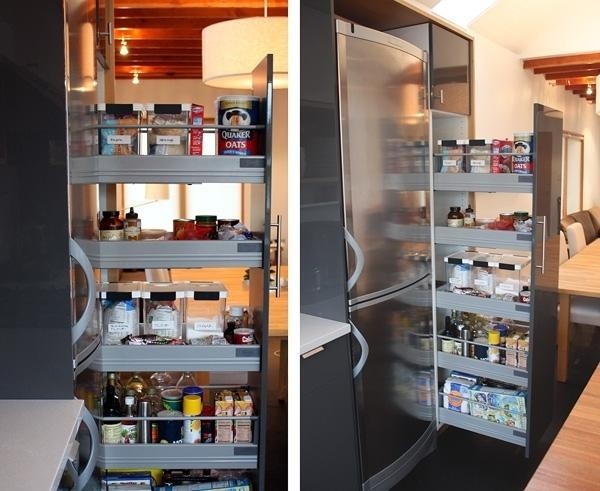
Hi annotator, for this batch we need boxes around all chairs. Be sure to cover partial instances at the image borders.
[559,203,600,382]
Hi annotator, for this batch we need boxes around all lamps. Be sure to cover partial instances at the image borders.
[202,0,289,90]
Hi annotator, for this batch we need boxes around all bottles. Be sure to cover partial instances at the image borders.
[500,212,527,231]
[103,373,213,442]
[101,207,141,241]
[442,311,508,366]
[195,215,216,239]
[447,204,477,227]
[228,306,254,345]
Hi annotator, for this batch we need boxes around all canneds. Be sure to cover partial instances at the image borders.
[173,219,194,240]
[234,329,254,344]
[514,213,528,220]
[218,219,239,231]
[500,213,512,222]
[196,216,217,232]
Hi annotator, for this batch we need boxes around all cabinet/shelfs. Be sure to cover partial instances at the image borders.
[432,102,562,460]
[71,52,274,491]
